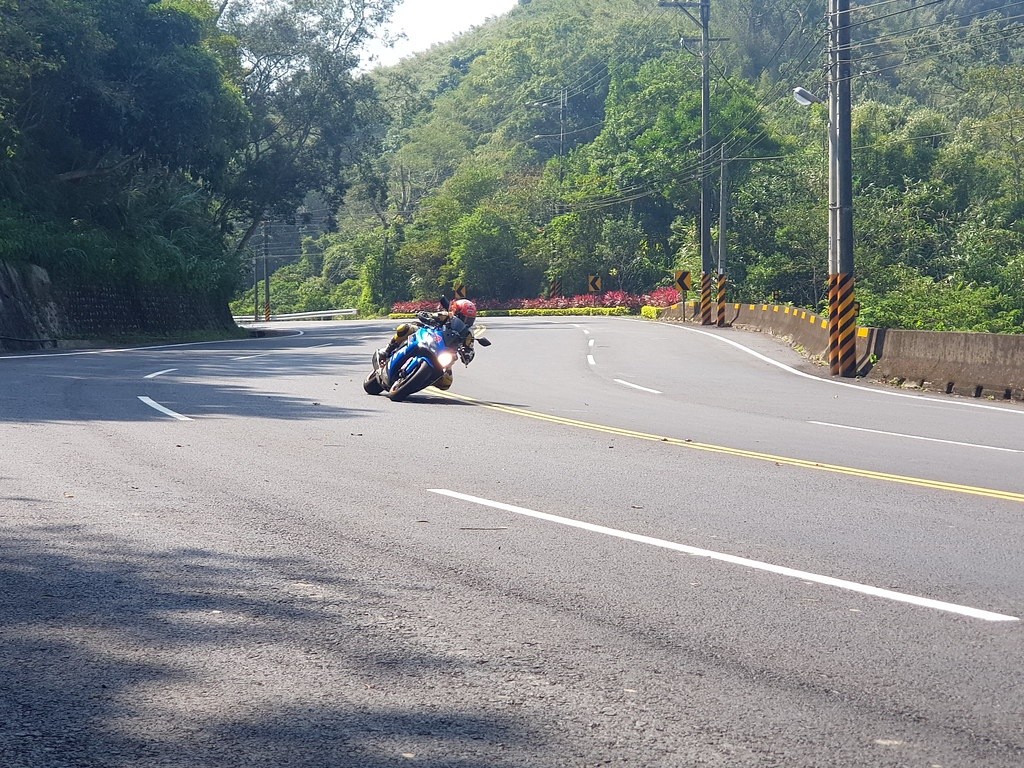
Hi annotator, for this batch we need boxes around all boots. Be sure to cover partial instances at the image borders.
[379,338,401,359]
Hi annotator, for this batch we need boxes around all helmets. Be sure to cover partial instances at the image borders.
[448,298,476,327]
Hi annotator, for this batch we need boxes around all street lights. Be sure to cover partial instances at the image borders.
[792,87,839,378]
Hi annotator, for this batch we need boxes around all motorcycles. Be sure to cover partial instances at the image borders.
[363,294,491,402]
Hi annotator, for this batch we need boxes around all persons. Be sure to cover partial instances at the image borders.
[380,298,478,390]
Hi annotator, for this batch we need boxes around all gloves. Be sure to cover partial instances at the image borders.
[461,351,473,364]
[417,310,431,322]
